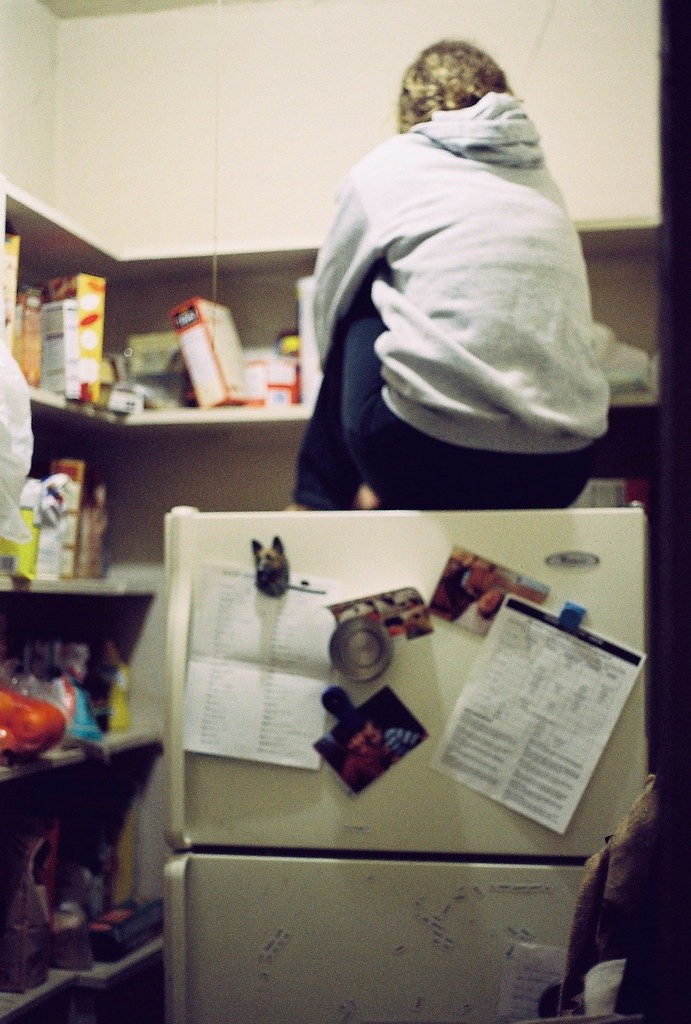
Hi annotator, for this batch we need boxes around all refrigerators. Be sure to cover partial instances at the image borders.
[146,503,654,1024]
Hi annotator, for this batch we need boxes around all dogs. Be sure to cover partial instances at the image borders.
[252,536,288,596]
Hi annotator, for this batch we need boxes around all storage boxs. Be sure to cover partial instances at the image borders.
[88,895,164,964]
[169,296,252,409]
[50,455,86,578]
[43,273,107,402]
[40,298,83,400]
[0,233,21,350]
[572,479,652,517]
[243,357,301,406]
[12,286,41,388]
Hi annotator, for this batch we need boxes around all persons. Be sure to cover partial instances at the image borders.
[289,42,613,510]
[442,564,503,635]
[339,719,424,792]
[0,339,35,543]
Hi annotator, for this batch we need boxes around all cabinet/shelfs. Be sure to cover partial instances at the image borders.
[0,181,669,1024]
